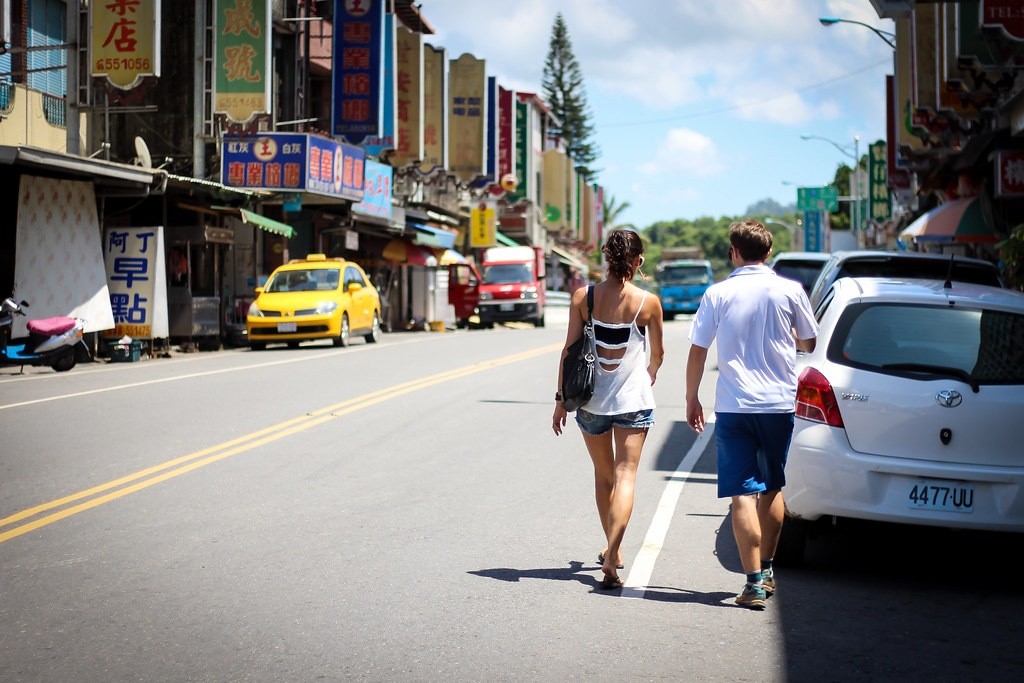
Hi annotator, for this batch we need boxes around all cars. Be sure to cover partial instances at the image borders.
[776,277,1024,609]
[769,251,832,298]
[246,253,382,350]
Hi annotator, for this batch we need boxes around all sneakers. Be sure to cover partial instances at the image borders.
[736,584,766,608]
[763,570,776,594]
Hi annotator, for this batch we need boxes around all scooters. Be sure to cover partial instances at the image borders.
[0,290,92,375]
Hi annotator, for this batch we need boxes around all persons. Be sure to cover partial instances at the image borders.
[552,230,664,588]
[686,218,821,609]
[327,272,339,288]
[165,245,182,291]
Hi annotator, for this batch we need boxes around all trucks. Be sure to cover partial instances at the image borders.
[448,246,547,329]
[654,260,714,322]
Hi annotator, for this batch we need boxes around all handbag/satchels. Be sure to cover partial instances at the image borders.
[562,285,595,412]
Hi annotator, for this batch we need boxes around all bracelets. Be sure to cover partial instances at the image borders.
[554,393,562,401]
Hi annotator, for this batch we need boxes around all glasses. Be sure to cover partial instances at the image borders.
[729,247,732,261]
[639,255,644,267]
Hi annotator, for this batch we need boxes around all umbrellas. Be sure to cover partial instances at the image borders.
[897,197,1024,244]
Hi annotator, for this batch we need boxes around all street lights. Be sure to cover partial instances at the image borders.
[801,133,863,250]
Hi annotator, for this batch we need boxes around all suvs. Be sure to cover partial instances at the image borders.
[808,249,1006,316]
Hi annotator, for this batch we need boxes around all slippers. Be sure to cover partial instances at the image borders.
[600,576,621,589]
[598,550,624,569]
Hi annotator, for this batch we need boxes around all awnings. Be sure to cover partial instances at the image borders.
[0,143,256,206]
[209,206,299,240]
[406,225,457,250]
